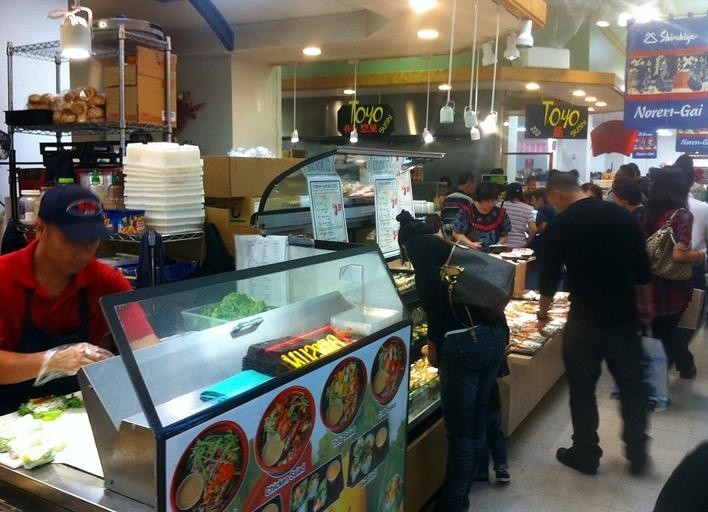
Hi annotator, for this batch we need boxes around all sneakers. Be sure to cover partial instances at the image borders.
[495,469,510,482]
[472,475,489,483]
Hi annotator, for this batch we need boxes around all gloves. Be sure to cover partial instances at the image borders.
[33,343,115,387]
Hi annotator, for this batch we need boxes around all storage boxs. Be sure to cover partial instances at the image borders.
[201,156,308,198]
[106,46,179,129]
[205,198,304,257]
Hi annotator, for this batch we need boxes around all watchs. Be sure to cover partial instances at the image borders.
[536,312,549,320]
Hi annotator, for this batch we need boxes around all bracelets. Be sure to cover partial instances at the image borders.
[697,248,707,264]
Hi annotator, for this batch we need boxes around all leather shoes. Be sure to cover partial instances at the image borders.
[679,365,696,381]
[625,435,652,469]
[557,443,603,476]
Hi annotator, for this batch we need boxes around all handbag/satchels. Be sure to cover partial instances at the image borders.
[403,235,516,338]
[635,209,693,281]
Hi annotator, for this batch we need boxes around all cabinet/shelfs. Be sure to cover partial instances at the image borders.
[386,257,565,512]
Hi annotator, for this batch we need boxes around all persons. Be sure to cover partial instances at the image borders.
[524,190,558,231]
[396,212,507,512]
[675,154,707,199]
[441,174,472,232]
[535,170,655,474]
[0,186,161,414]
[612,178,671,412]
[472,329,510,483]
[498,182,535,249]
[667,164,707,251]
[643,165,708,379]
[449,184,510,252]
[610,161,648,204]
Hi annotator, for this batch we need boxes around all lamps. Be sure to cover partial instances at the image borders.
[440,0,457,124]
[515,20,534,49]
[463,0,479,129]
[290,62,300,143]
[482,41,498,66]
[422,71,434,144]
[349,58,358,144]
[470,45,481,141]
[503,35,520,61]
[482,8,500,134]
[46,0,99,61]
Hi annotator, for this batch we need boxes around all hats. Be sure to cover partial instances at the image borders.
[38,184,111,242]
[506,183,522,194]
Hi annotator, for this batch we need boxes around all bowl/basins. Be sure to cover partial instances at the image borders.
[502,256,519,262]
[181,303,231,330]
[593,179,613,188]
[123,142,206,237]
[253,386,316,478]
[3,109,54,126]
[518,254,530,260]
[489,245,507,252]
[170,421,249,512]
[320,357,368,433]
[371,337,407,405]
